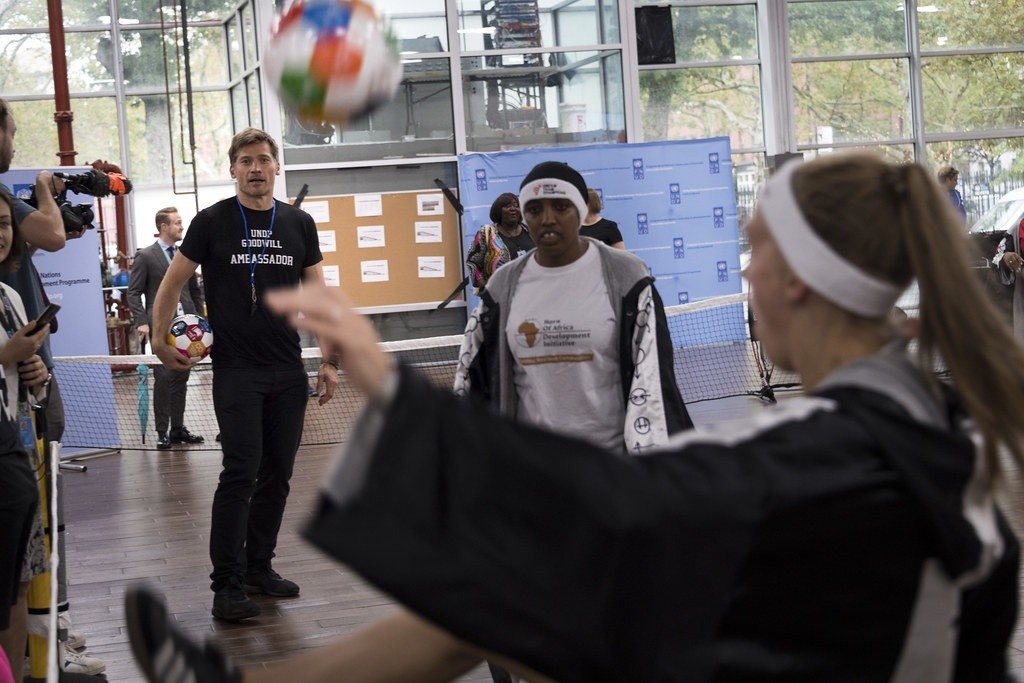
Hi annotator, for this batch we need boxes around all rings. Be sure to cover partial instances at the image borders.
[1009,261,1012,263]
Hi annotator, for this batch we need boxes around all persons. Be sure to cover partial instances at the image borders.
[150,128,340,620]
[453,161,697,454]
[125,206,205,449]
[578,188,625,249]
[0,99,107,683]
[465,193,537,296]
[119,148,1024,683]
[937,166,967,220]
[991,212,1024,342]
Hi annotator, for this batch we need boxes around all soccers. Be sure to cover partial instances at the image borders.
[166,313,214,363]
[264,0,405,125]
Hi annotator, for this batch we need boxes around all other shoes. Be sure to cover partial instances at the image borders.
[216,435,222,441]
[308,386,319,397]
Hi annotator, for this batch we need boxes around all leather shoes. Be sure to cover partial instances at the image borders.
[156,431,171,448]
[169,426,204,443]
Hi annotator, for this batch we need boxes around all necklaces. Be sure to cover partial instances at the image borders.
[235,194,276,302]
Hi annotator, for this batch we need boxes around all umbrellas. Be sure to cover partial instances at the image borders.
[135,333,150,444]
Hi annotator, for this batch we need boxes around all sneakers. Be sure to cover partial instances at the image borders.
[243,570,299,596]
[211,593,265,621]
[20,655,33,676]
[64,633,87,649]
[125,581,243,683]
[57,639,107,674]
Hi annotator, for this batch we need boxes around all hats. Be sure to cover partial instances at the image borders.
[520,161,589,230]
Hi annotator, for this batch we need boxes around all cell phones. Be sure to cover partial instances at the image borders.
[25,303,61,336]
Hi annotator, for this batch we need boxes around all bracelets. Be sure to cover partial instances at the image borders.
[321,358,340,371]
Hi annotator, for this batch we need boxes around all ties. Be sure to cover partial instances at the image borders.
[166,246,175,261]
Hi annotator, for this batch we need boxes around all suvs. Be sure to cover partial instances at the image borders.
[968,186,1024,330]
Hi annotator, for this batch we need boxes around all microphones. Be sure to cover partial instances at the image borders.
[52,172,133,198]
